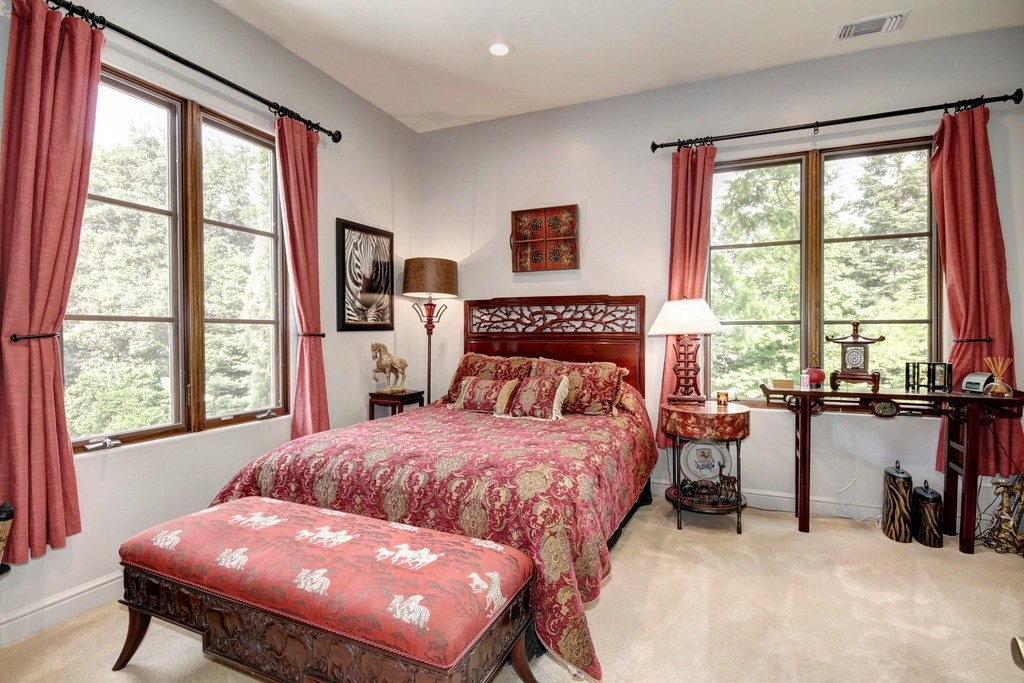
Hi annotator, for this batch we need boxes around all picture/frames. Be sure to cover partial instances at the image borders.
[336,218,394,331]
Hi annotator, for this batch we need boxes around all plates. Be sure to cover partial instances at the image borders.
[681,440,732,485]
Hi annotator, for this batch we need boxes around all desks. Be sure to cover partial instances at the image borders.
[368,390,425,420]
[760,383,1024,554]
[658,402,751,531]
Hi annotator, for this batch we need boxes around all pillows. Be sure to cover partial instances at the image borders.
[536,356,630,416]
[511,375,569,421]
[453,376,518,414]
[448,352,539,406]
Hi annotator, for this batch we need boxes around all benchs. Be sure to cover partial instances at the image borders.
[111,494,539,683]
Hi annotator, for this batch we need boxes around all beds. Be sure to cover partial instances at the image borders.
[204,295,658,683]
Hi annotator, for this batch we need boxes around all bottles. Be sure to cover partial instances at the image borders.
[800,370,810,391]
[985,377,1014,396]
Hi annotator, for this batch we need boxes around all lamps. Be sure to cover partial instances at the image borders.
[402,257,458,405]
[647,296,724,406]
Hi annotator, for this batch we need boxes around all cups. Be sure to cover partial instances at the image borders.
[718,391,728,407]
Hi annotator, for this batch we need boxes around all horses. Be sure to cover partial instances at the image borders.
[369,340,409,388]
